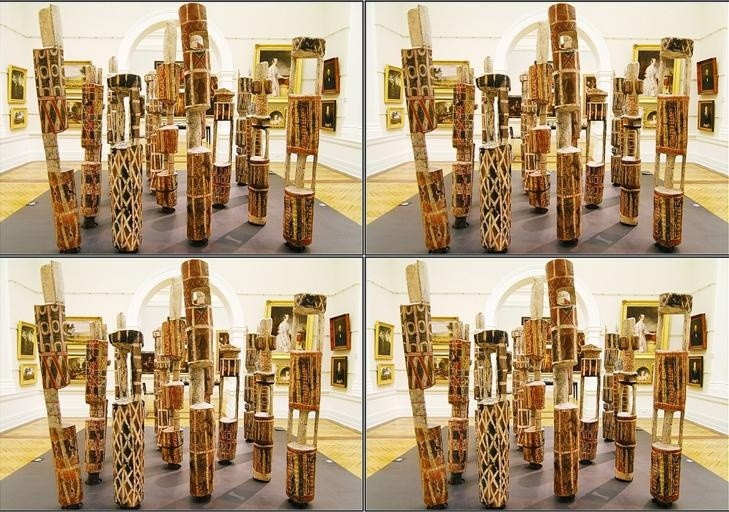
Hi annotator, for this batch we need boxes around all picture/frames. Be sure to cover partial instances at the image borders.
[375,316,462,387]
[266,299,352,390]
[18,317,104,385]
[251,44,340,131]
[7,60,93,130]
[617,300,707,387]
[384,61,475,130]
[631,44,718,132]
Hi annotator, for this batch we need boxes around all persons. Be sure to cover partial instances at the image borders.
[690,361,700,384]
[336,323,346,345]
[637,369,648,381]
[701,104,712,128]
[21,330,33,354]
[276,314,292,351]
[324,105,331,127]
[334,361,343,383]
[691,324,703,347]
[12,73,23,99]
[702,68,713,90]
[324,68,334,88]
[643,58,658,97]
[378,330,390,355]
[268,56,288,97]
[282,369,291,381]
[633,313,655,353]
